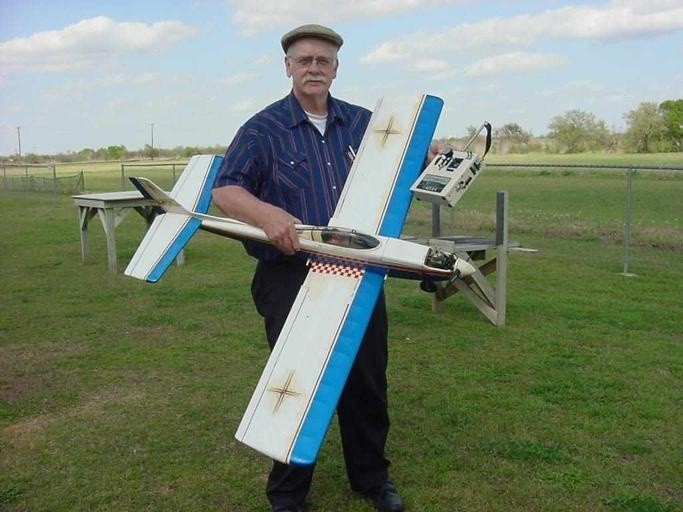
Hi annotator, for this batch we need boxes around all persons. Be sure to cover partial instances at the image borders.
[205,23,463,511]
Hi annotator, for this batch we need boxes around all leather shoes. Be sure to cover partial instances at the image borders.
[367,475,404,512]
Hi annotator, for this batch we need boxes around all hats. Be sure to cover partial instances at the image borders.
[281,24,344,54]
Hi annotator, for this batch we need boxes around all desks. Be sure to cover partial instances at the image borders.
[71,191,185,273]
